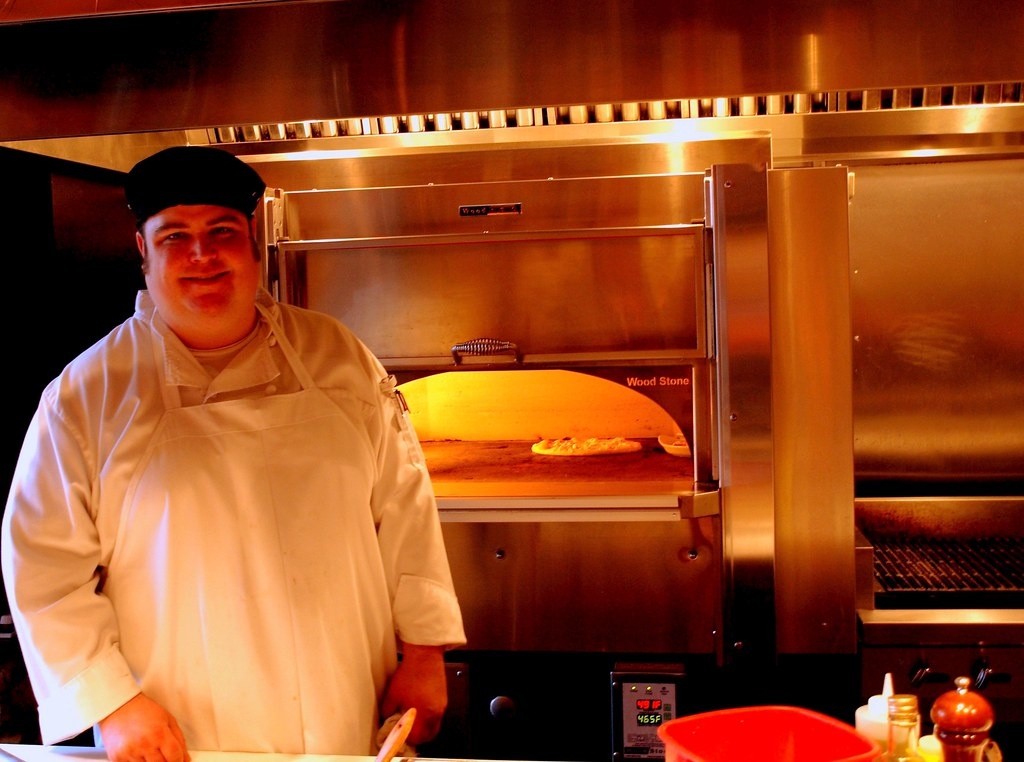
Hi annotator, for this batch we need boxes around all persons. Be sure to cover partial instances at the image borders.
[0,145,467,762]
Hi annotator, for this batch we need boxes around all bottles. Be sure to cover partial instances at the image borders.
[855,672,1024,762]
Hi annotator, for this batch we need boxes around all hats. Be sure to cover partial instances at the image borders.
[124,146,267,227]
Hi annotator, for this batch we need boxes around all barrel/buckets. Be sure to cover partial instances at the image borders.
[658,705,879,762]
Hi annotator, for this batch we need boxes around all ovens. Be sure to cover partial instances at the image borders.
[277,169,723,760]
